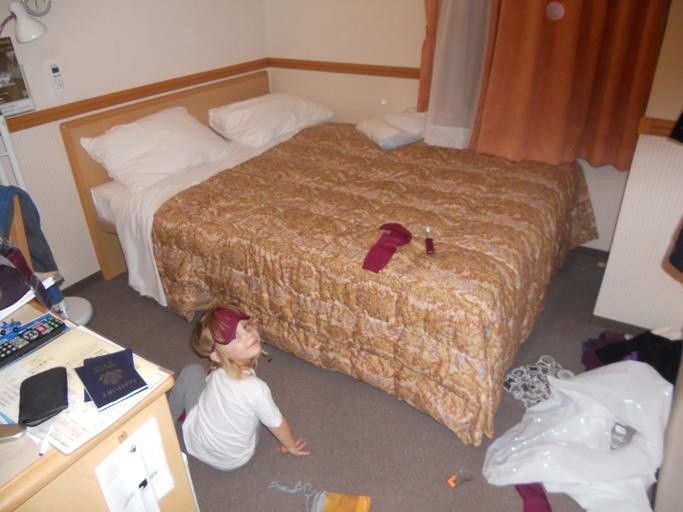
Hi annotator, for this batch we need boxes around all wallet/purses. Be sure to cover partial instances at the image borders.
[18,367,68,427]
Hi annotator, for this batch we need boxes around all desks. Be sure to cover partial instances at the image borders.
[0,300,199,512]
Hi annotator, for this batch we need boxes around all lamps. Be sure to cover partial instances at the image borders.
[0,1,48,44]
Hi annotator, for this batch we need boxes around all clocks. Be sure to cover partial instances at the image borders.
[22,0,50,18]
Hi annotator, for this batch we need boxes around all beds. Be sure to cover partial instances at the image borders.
[58,65,599,447]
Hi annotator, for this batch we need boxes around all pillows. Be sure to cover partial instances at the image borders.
[208,90,337,151]
[78,105,231,194]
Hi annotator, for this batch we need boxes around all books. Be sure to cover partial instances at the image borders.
[74,348,149,412]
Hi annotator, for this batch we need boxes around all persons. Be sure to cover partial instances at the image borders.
[168,304,311,472]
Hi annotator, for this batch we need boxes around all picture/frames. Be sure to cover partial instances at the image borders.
[0,36,34,121]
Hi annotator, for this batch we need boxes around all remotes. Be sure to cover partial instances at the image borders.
[0,316,65,368]
